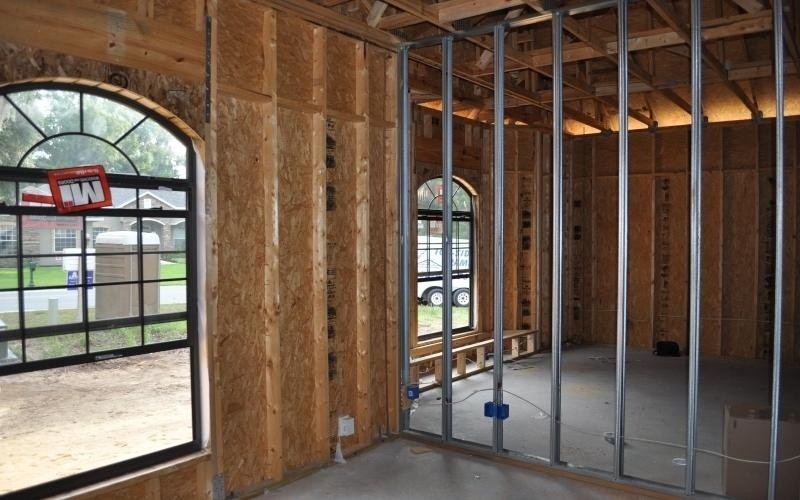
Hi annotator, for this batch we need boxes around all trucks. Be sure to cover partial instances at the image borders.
[416,238,471,306]
